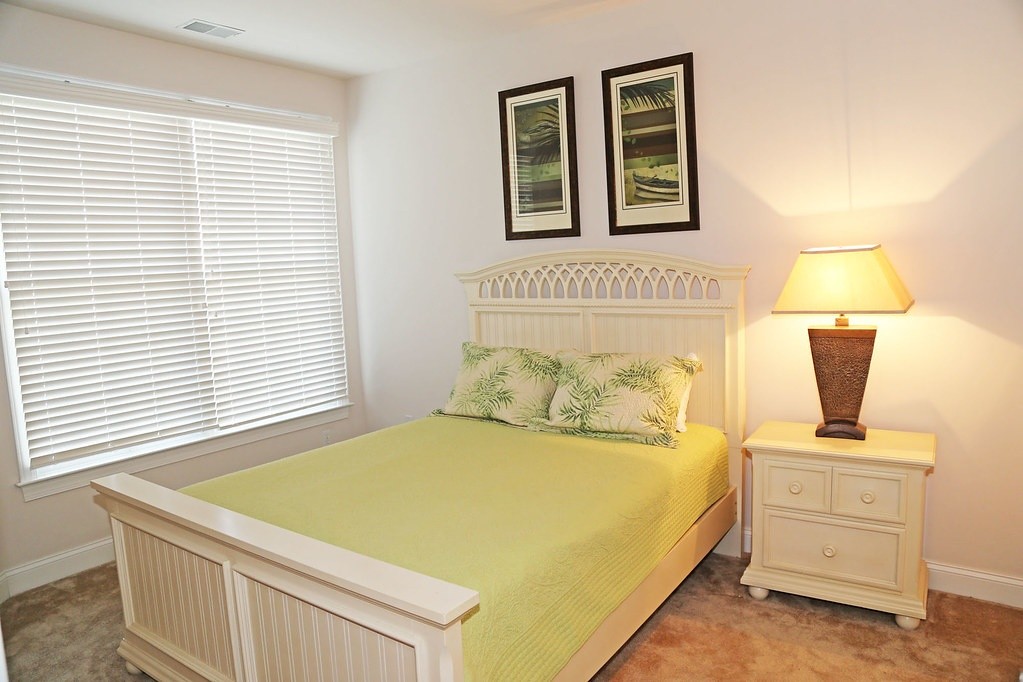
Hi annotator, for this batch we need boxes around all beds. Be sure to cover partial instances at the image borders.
[87,247,751,682]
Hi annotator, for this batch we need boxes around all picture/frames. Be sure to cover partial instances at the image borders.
[600,52,701,238]
[496,75,581,243]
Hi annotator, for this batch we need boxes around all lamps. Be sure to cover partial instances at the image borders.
[767,244,916,441]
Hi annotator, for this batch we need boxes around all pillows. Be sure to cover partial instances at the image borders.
[428,341,565,431]
[529,351,703,450]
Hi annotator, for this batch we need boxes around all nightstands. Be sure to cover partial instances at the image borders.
[739,418,938,629]
